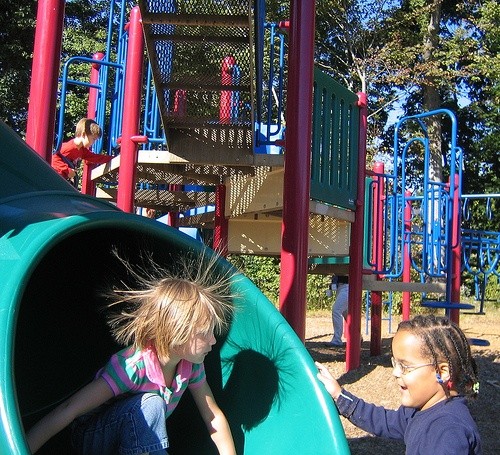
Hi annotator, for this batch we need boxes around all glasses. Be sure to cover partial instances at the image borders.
[391,356,442,374]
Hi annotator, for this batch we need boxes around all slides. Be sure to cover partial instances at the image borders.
[0,116,349,455]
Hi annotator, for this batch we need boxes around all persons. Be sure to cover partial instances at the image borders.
[315,315,484,455]
[324,275,363,348]
[51,118,116,179]
[24,236,247,455]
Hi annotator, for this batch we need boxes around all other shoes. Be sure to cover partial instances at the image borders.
[324,342,344,348]
